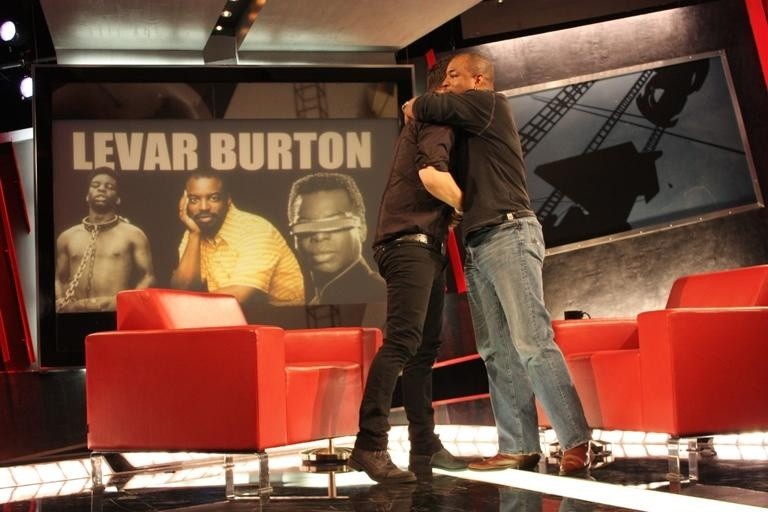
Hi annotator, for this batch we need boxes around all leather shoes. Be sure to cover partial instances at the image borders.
[559,440,596,475]
[468,453,542,470]
[348,448,418,485]
[410,445,467,471]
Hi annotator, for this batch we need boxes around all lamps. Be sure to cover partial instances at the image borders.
[20,76,34,99]
[0,21,21,46]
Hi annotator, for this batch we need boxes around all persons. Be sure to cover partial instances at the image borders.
[55,167,156,313]
[170,166,304,308]
[287,173,388,306]
[403,51,595,477]
[347,58,464,484]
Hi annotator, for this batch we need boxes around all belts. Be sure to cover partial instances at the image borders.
[371,233,447,255]
[463,210,537,244]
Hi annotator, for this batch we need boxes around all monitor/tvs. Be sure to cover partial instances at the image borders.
[33,63,416,371]
[498,47,764,258]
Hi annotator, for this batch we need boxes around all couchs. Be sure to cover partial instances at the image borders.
[537,265,768,493]
[85,289,382,502]
